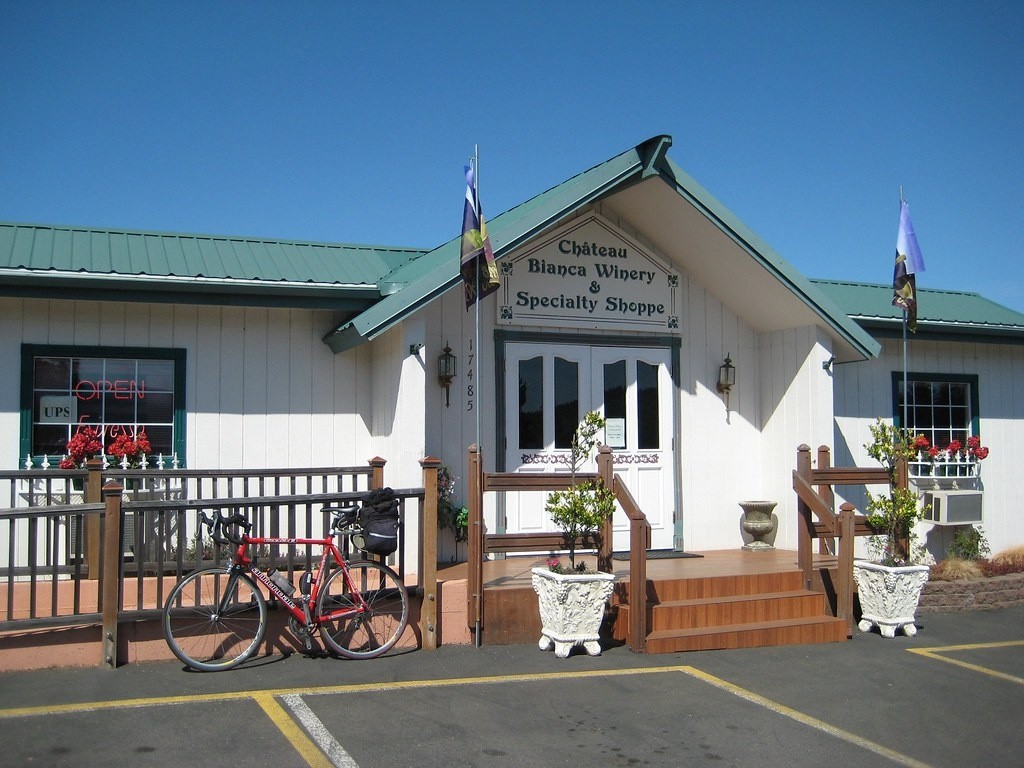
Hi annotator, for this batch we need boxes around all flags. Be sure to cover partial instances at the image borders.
[893,199,924,335]
[459,159,500,312]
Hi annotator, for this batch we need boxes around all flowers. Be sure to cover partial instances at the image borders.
[879,542,907,567]
[60,429,101,469]
[934,437,989,460]
[547,557,560,572]
[109,432,150,469]
[903,437,934,465]
[438,466,470,562]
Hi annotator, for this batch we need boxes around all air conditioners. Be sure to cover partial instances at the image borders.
[923,489,985,526]
[68,513,147,559]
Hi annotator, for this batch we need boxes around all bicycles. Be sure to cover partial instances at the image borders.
[161,504,409,673]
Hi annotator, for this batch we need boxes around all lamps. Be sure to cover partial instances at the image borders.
[439,341,457,410]
[716,352,736,395]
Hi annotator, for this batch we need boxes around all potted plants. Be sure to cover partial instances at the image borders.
[529,406,616,660]
[853,416,929,637]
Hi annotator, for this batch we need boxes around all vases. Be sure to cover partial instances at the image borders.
[917,466,928,476]
[739,501,778,551]
[74,476,86,491]
[952,464,974,475]
[119,473,143,490]
